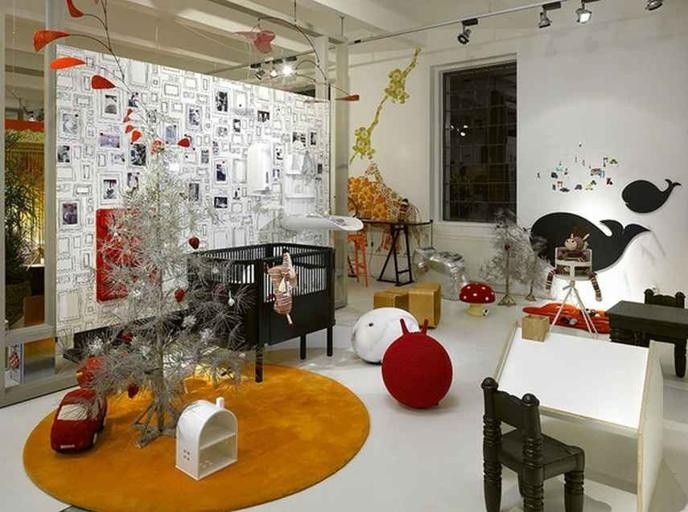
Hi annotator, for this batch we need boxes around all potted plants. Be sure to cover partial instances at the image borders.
[0,133,38,325]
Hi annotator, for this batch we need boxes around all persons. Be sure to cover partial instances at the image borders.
[132,92,139,107]
[131,143,146,164]
[293,132,305,147]
[57,144,71,163]
[63,203,78,225]
[128,94,138,106]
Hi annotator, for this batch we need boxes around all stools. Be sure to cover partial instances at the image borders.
[372,282,499,330]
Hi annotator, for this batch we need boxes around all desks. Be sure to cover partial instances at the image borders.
[602,301,686,378]
[344,217,435,286]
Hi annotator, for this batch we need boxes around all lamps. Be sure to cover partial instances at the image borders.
[271,66,278,78]
[645,0,664,12]
[458,25,470,45]
[282,60,294,76]
[575,0,592,22]
[254,65,265,80]
[538,9,553,28]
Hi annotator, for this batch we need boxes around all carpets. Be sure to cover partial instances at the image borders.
[24,360,370,511]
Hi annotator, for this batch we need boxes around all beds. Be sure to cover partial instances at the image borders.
[187,246,336,382]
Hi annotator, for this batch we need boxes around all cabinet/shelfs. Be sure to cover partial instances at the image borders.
[496,322,664,511]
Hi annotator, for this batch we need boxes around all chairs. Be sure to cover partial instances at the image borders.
[478,373,585,512]
[642,287,686,309]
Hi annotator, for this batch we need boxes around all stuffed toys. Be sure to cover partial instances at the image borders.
[267,247,296,328]
[544,232,603,303]
